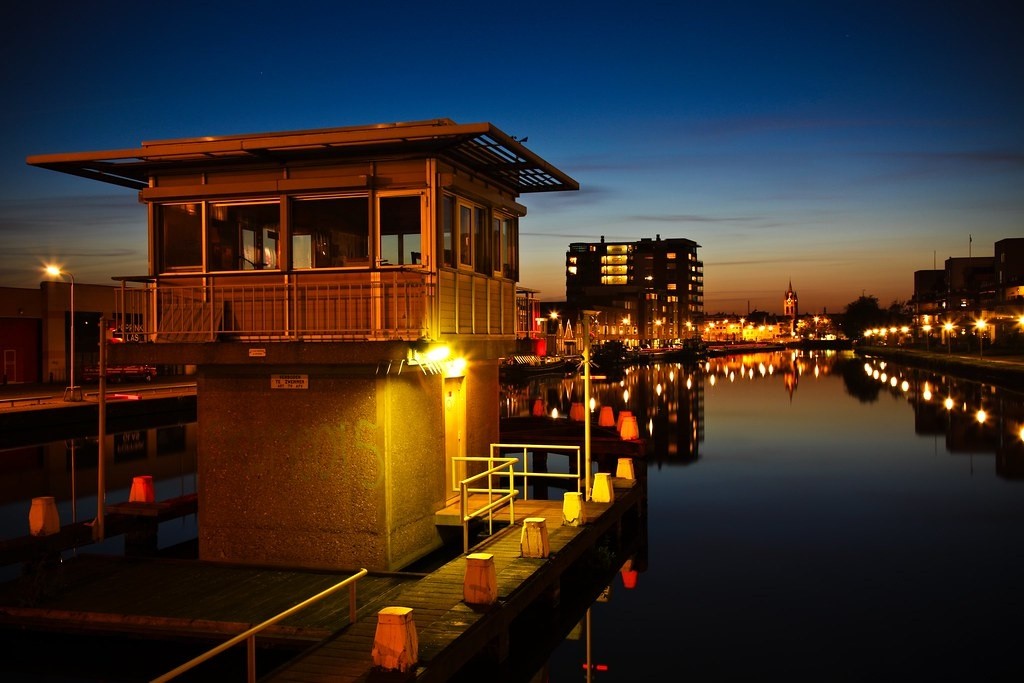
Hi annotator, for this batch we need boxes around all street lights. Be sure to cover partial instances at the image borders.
[889,328,898,346]
[978,398,985,423]
[686,322,692,337]
[945,381,952,409]
[45,266,76,401]
[899,327,907,349]
[923,326,931,352]
[944,322,954,354]
[976,321,984,356]
[740,318,744,341]
